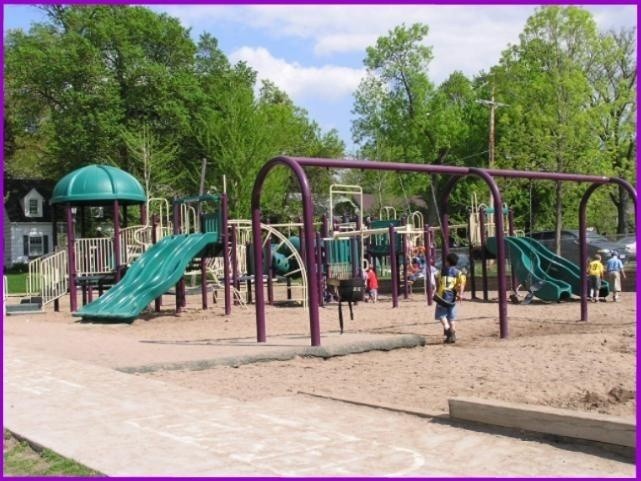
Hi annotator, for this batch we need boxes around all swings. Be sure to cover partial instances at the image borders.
[507,179,534,306]
[397,170,460,309]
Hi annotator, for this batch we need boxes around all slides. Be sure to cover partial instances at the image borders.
[71,232,218,320]
[486,232,609,303]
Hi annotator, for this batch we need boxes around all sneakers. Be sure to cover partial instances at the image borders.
[442,329,458,344]
[589,296,620,303]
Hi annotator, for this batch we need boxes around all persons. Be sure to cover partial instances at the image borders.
[410,257,421,282]
[367,264,379,305]
[432,252,467,343]
[586,254,604,303]
[607,251,628,302]
[423,259,439,298]
